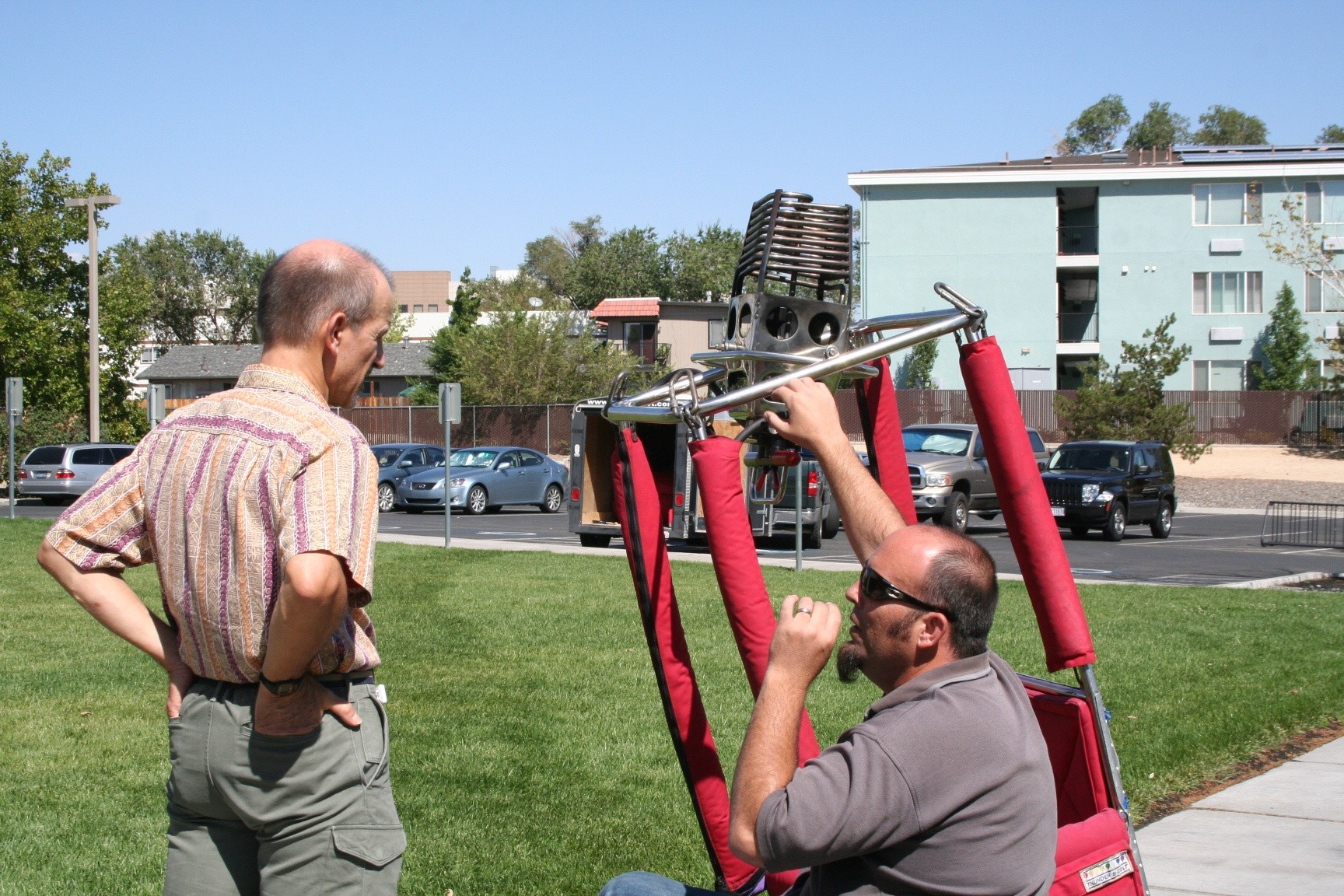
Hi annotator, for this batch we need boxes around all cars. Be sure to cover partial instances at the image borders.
[398,446,569,514]
[370,443,462,512]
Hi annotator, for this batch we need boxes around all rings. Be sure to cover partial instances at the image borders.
[796,609,811,617]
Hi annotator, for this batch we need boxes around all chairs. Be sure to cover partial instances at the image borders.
[434,455,444,463]
[386,454,398,464]
[525,459,538,465]
[407,453,421,467]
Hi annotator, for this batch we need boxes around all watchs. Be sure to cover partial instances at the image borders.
[259,668,306,696]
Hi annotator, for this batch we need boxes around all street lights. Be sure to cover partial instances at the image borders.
[65,193,120,445]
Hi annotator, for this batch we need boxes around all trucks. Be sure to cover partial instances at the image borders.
[567,395,755,548]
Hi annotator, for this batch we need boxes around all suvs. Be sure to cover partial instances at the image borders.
[862,423,1050,537]
[18,442,139,505]
[746,433,840,547]
[1038,439,1177,542]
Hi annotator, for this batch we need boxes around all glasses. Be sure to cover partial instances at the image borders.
[860,559,957,623]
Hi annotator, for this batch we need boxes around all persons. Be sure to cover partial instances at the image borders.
[35,239,406,896]
[599,374,1057,896]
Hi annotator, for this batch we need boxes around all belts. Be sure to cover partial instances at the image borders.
[195,668,375,687]
